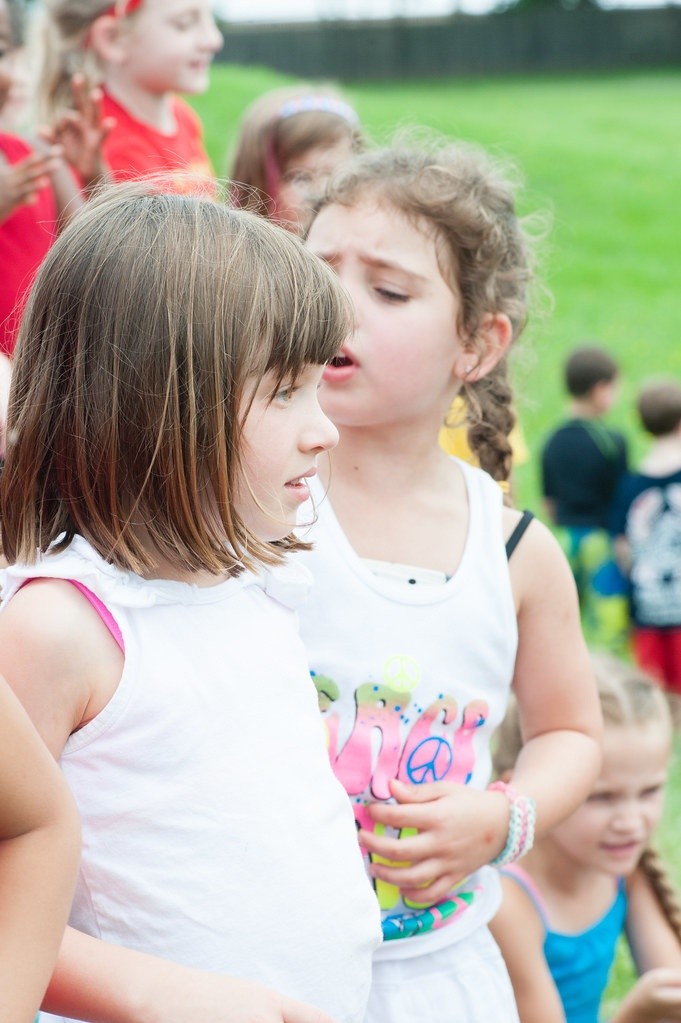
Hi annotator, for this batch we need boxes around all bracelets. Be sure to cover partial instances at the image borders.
[490,781,536,867]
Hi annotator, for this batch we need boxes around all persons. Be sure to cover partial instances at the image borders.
[543,345,681,691]
[222,118,557,1023]
[1,1,378,458]
[1,171,381,1023]
[486,656,681,1023]
[0,672,82,1023]
